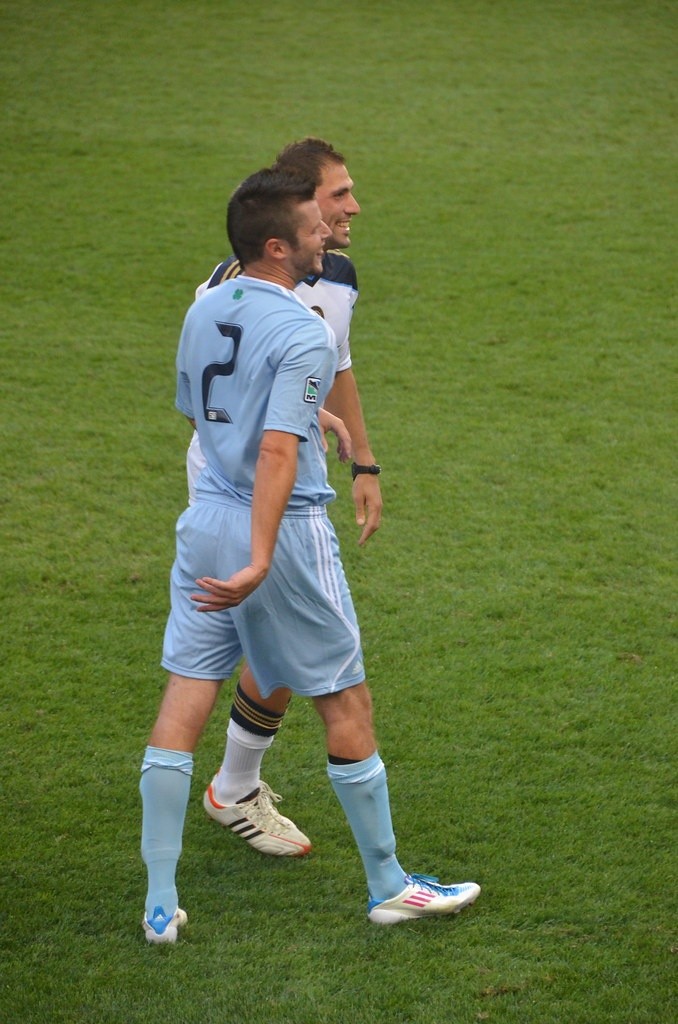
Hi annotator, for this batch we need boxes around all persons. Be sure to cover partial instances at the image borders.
[138,170,481,944]
[185,139,381,857]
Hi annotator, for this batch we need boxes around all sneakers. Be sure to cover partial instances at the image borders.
[141,905,188,945]
[366,873,481,926]
[203,773,312,857]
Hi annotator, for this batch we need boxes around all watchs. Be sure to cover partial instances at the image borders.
[351,462,381,481]
